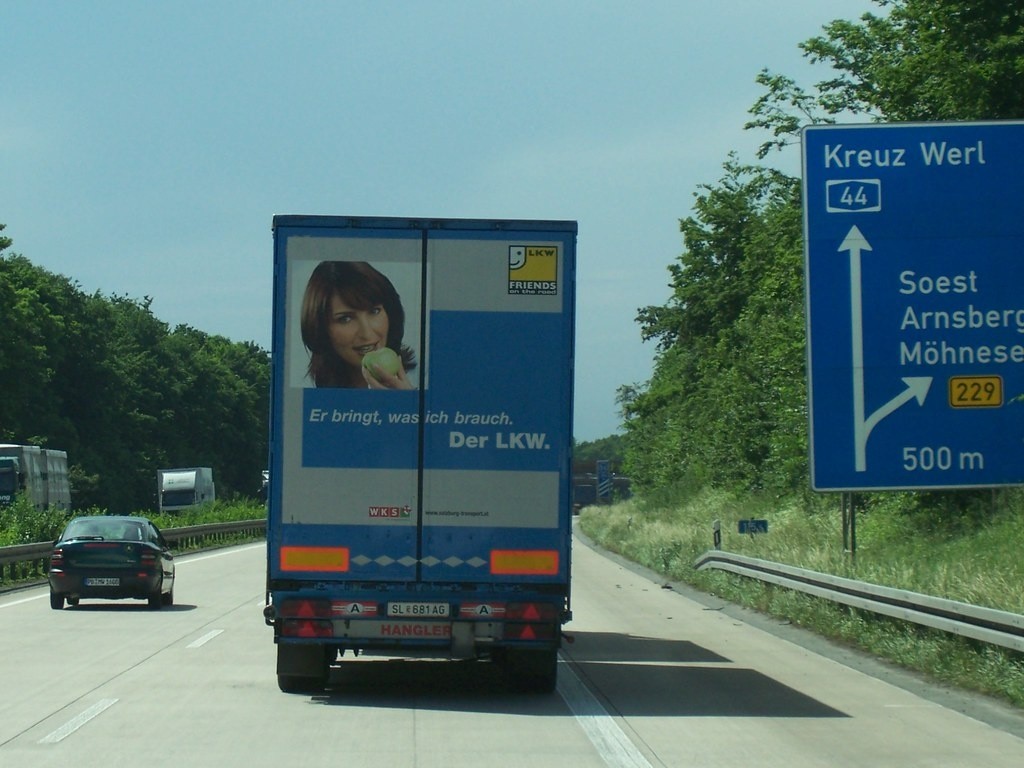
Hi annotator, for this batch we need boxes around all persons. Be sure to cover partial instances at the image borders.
[301,262,420,390]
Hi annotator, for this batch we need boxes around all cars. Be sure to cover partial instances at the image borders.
[46,515,178,610]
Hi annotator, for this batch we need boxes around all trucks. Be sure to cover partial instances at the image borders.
[256,469,269,505]
[259,213,581,694]
[156,466,217,516]
[0,443,73,513]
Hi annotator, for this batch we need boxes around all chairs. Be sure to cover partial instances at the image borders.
[122,527,140,541]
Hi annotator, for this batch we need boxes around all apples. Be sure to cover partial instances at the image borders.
[362,348,400,382]
